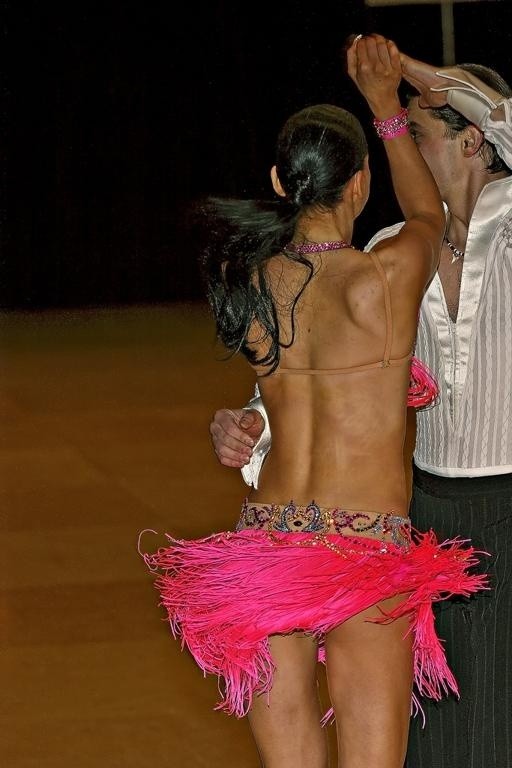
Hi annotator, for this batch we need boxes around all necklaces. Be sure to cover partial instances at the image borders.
[287,240,355,253]
[446,237,464,287]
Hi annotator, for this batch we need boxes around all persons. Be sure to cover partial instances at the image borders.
[207,28,511,768]
[135,32,494,766]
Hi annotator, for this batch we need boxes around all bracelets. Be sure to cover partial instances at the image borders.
[373,110,410,141]
